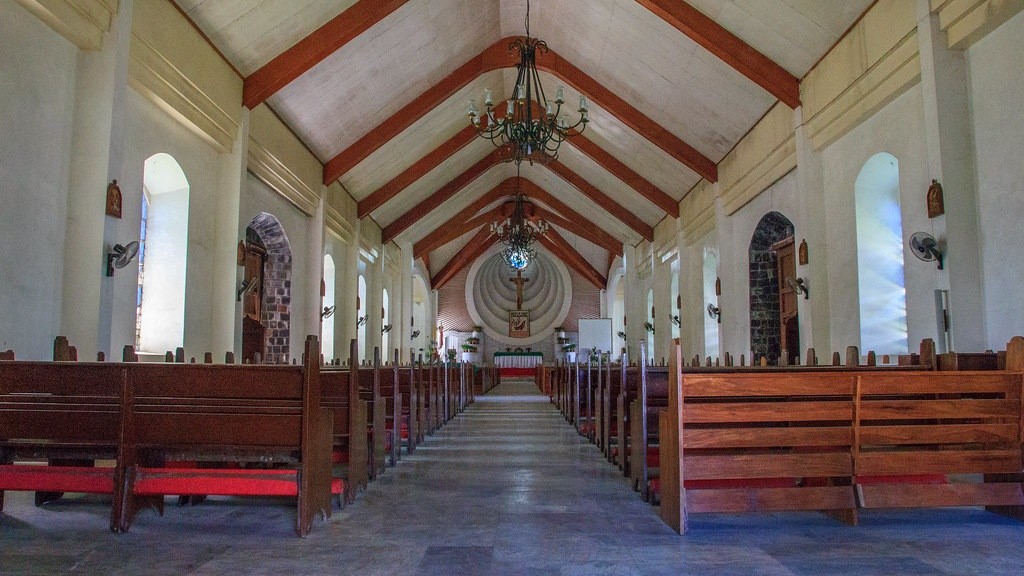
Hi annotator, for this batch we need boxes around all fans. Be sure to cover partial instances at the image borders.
[617,331,627,341]
[644,322,655,335]
[707,303,721,323]
[320,306,336,323]
[356,314,369,330]
[381,324,392,335]
[668,313,682,328]
[237,276,260,301]
[411,330,420,340]
[909,232,943,270]
[106,241,139,277]
[788,277,808,300]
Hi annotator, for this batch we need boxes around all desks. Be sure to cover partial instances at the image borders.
[494,351,544,368]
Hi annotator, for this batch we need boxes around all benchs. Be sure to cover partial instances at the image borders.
[534,338,1024,536]
[0,334,501,540]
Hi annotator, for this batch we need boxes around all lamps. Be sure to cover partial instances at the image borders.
[490,164,549,251]
[500,244,537,272]
[467,0,589,166]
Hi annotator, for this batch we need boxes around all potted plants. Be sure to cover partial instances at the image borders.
[462,345,469,352]
[465,337,473,344]
[472,337,480,344]
[467,346,475,352]
[564,337,570,344]
[472,325,483,332]
[569,344,577,352]
[562,346,569,352]
[554,327,566,338]
